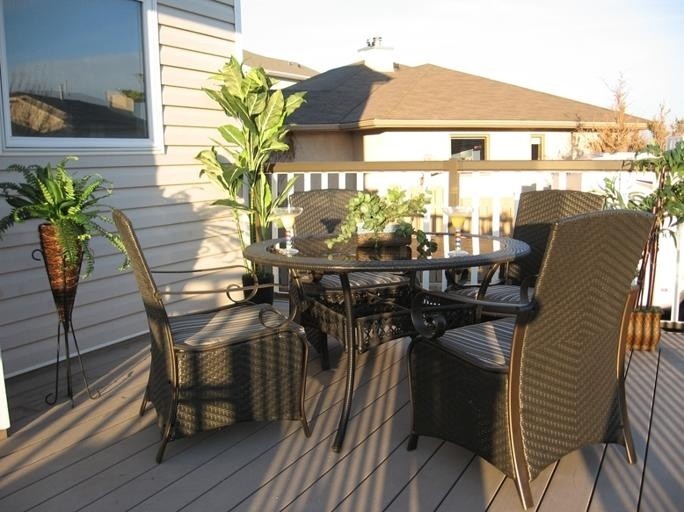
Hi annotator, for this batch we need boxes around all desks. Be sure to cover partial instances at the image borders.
[239,221,533,458]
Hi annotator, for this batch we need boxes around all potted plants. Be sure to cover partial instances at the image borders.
[579,135,684,353]
[0,142,131,335]
[189,52,309,309]
[321,182,441,256]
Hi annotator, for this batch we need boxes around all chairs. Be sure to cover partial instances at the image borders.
[108,202,315,462]
[280,185,418,370]
[435,185,607,328]
[399,207,658,510]
